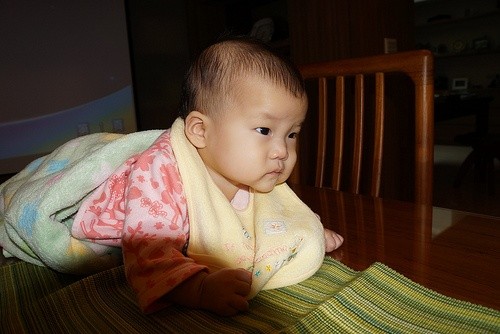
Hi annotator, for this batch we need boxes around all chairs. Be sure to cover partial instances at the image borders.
[289,49,434,207]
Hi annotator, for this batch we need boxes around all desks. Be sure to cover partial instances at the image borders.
[0,182,500,334]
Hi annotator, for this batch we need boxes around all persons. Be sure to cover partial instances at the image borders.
[72,39,345,317]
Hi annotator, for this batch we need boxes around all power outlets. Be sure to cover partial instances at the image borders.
[383,38,398,54]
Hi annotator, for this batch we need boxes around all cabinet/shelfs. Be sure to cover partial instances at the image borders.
[416,11,500,96]
